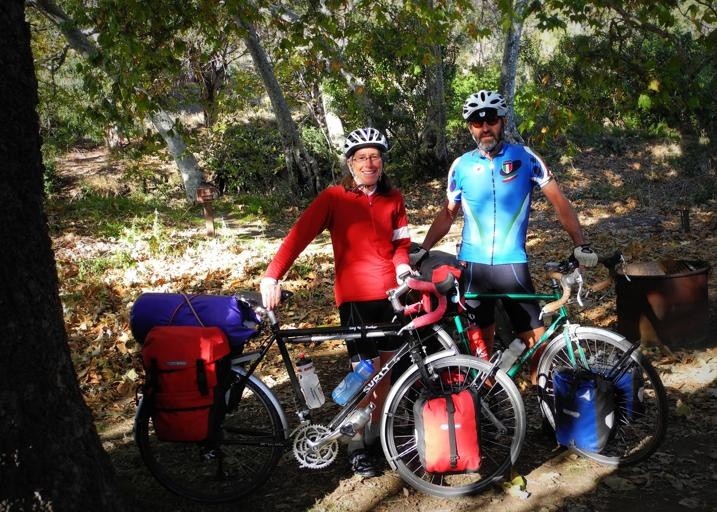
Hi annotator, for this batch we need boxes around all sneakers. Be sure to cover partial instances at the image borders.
[347,448,374,478]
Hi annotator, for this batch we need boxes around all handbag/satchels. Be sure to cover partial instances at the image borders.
[413,388,483,473]
[552,352,644,452]
[142,325,231,442]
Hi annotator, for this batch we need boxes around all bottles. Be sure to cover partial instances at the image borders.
[293,353,326,411]
[329,357,377,408]
[466,322,490,362]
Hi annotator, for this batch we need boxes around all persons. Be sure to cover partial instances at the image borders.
[259,127,419,479]
[407,88,599,433]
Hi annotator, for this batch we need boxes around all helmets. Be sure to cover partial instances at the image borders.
[344,129,389,158]
[461,89,510,123]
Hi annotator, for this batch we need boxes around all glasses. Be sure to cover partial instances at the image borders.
[471,121,500,128]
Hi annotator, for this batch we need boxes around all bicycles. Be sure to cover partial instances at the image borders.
[128,249,528,505]
[403,250,668,467]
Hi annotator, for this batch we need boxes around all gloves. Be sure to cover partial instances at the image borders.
[409,247,429,271]
[573,244,599,268]
